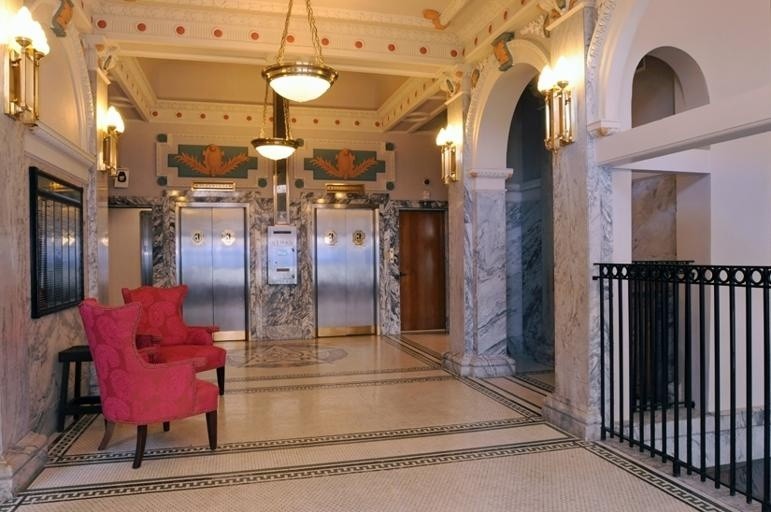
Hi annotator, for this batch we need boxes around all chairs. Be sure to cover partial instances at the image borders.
[78,298,219,469]
[122,285,227,395]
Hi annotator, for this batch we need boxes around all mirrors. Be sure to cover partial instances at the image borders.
[29,166,85,319]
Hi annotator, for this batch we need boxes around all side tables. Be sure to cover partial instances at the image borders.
[57,345,110,431]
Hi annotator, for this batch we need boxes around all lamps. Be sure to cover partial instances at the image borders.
[537,55,574,151]
[435,126,461,185]
[4,5,50,130]
[251,80,297,162]
[261,1,338,103]
[104,106,125,177]
[60,227,76,246]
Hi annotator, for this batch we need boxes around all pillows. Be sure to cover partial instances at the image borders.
[137,301,189,347]
